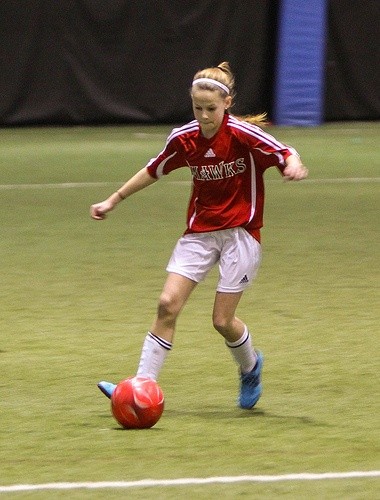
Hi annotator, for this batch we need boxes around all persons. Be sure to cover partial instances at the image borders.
[90,62,310,411]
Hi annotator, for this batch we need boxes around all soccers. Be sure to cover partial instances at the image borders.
[112,377,163,430]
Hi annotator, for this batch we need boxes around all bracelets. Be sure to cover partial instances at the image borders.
[116,191,125,202]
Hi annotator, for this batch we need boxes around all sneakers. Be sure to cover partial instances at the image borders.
[239,354,263,409]
[98,381,117,400]
